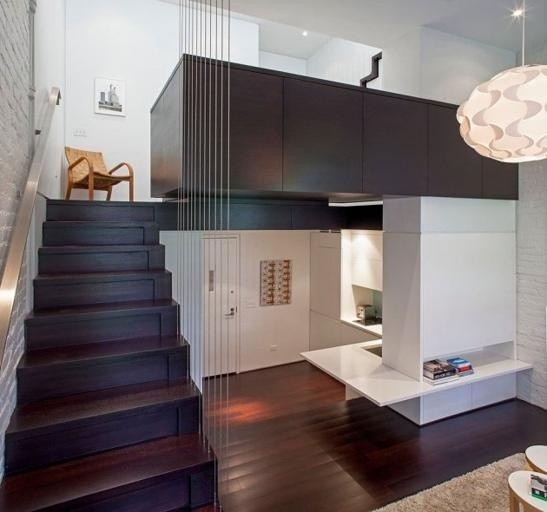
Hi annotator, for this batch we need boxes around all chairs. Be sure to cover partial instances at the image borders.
[65,146,133,201]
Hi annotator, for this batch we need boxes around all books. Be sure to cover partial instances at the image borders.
[422,357,474,386]
[530,473,547,500]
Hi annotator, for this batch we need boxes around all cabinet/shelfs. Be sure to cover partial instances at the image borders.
[311,231,517,426]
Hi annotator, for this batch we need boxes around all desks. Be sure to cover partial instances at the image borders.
[526,445,546,474]
[507,469,546,512]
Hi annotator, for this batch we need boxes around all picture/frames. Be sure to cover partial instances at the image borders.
[95,78,126,116]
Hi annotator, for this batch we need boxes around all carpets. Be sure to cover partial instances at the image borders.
[370,452,534,512]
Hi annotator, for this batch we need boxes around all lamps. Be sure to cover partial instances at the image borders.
[456,2,547,162]
